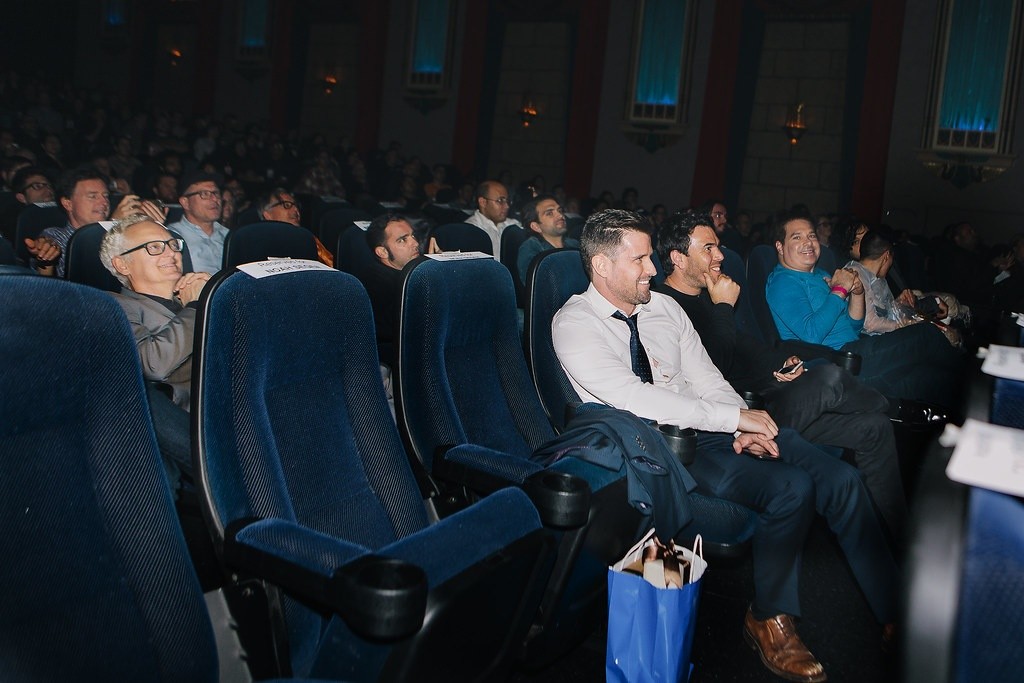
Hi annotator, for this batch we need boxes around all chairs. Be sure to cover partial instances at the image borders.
[0,192,841,683]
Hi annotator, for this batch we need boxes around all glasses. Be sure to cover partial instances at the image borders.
[22,182,53,192]
[186,190,221,199]
[483,196,513,206]
[269,200,298,209]
[119,237,185,256]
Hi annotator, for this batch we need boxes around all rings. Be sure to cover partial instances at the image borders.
[51,244,58,249]
[45,240,52,246]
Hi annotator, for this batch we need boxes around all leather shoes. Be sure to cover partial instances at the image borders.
[890,399,950,428]
[741,602,828,683]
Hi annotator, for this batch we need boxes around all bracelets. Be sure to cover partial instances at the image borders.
[34,256,60,269]
[832,285,849,297]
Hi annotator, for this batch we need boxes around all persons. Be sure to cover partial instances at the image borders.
[0,105,1024,682]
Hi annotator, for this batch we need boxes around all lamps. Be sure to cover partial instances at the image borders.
[325,66,345,93]
[518,97,537,128]
[781,104,806,144]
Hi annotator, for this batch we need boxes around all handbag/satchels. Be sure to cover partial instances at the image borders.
[605,526,706,683]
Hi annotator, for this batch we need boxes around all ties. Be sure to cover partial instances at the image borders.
[610,309,655,385]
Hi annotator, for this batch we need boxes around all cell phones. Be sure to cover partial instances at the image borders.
[777,360,803,375]
[130,199,164,216]
